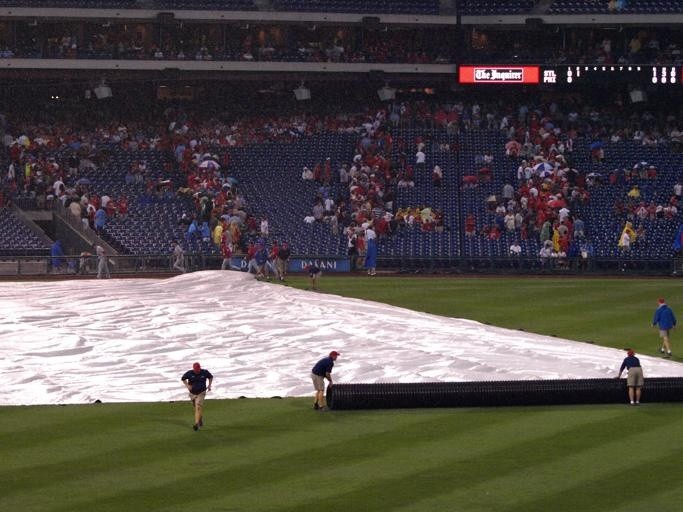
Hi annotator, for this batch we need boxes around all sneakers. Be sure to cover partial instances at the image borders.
[631,403,639,405]
[659,347,672,356]
[193,416,202,431]
[314,403,326,411]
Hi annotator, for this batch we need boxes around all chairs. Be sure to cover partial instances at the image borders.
[0,129,683,256]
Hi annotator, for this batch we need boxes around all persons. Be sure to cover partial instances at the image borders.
[1,18,682,67]
[311,350,340,411]
[619,350,645,406]
[0,83,683,290]
[651,297,676,356]
[182,363,213,431]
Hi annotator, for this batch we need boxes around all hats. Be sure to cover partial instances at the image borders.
[659,298,664,302]
[194,363,201,373]
[627,351,633,355]
[330,351,340,356]
